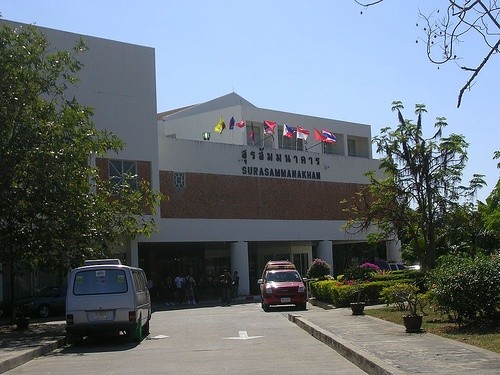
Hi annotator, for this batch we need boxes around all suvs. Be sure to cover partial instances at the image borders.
[258,260,308,311]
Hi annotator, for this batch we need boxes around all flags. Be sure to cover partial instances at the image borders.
[229,117,235,129]
[313,127,337,144]
[215,116,226,133]
[249,123,255,145]
[283,124,295,139]
[237,119,245,127]
[264,120,276,135]
[297,126,311,141]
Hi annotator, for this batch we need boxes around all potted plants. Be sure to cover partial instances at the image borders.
[350,280,366,315]
[401,292,424,333]
[15,312,30,330]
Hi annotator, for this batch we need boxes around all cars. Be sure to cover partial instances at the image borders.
[383,262,406,273]
[406,265,420,271]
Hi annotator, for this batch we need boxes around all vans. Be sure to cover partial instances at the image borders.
[64,258,152,343]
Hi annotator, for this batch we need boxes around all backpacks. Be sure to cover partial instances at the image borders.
[185,277,191,289]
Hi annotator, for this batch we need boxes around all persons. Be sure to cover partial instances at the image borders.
[164,272,201,306]
[206,268,239,307]
